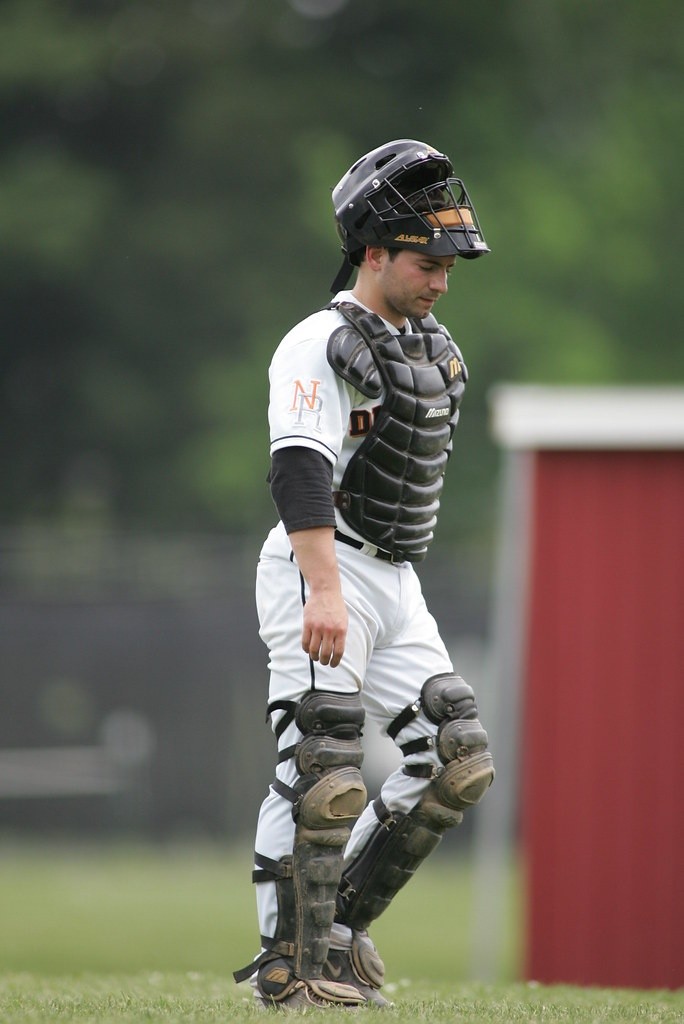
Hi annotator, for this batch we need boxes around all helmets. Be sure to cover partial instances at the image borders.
[332,138,487,259]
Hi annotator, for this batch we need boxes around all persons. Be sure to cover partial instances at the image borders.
[233,139,495,1011]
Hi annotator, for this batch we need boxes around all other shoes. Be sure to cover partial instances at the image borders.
[332,975,396,1012]
[256,985,362,1012]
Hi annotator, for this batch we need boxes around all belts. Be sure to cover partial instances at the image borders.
[335,530,404,565]
